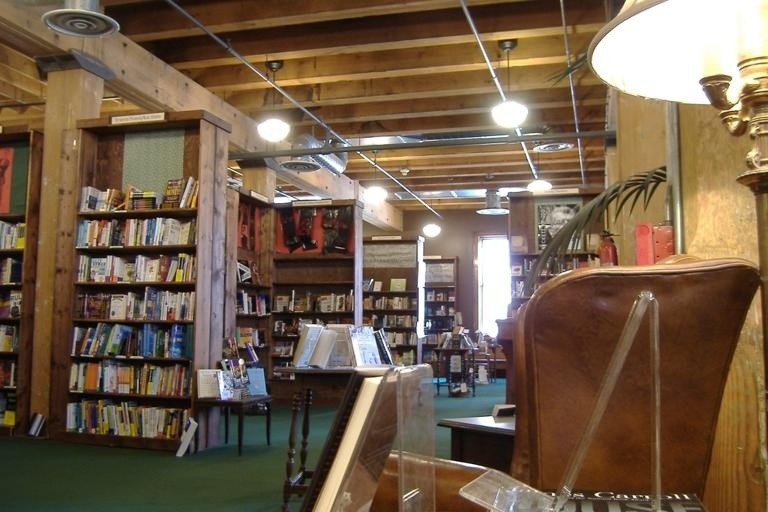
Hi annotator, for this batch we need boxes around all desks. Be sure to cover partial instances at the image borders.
[437,415,516,477]
[273,365,396,511]
[193,395,272,455]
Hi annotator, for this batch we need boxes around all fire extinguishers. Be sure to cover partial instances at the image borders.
[598,229,621,267]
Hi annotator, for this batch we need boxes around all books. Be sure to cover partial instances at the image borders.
[311,375,423,512]
[64,176,196,438]
[512,254,602,312]
[1,219,27,428]
[197,278,456,402]
[174,417,197,457]
[28,412,46,437]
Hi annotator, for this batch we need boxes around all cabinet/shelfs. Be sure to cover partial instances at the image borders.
[272,200,364,408]
[363,235,425,364]
[227,185,274,393]
[0,128,44,436]
[425,256,457,377]
[50,111,222,454]
[432,348,479,397]
[506,186,605,319]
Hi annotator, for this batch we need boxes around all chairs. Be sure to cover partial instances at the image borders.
[372,256,760,511]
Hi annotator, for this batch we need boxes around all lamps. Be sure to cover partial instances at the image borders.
[490,51,529,132]
[422,199,442,239]
[588,0,766,341]
[526,150,553,191]
[258,69,289,144]
[364,150,388,201]
